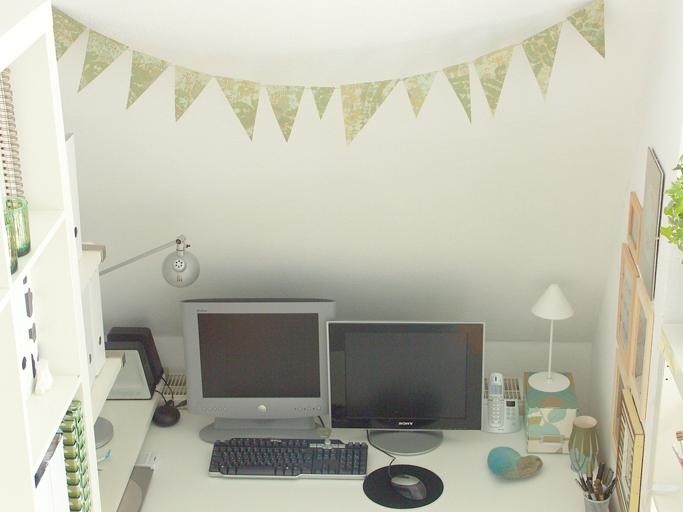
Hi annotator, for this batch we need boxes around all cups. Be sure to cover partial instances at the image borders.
[582,492,611,512]
[4,196,32,276]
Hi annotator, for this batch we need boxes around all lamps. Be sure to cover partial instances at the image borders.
[98,234,200,289]
[529,282,575,395]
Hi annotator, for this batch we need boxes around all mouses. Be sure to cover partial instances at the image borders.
[391,474,428,500]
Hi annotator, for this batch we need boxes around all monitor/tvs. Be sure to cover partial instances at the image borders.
[180,298,336,443]
[326,320,486,456]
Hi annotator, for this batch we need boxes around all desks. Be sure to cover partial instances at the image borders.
[119,405,611,512]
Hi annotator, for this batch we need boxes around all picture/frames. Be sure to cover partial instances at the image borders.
[615,243,637,390]
[612,367,645,512]
[625,191,642,264]
[629,277,654,423]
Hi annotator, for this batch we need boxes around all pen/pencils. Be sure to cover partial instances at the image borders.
[574,462,618,502]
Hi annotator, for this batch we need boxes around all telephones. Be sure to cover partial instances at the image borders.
[483,372,524,434]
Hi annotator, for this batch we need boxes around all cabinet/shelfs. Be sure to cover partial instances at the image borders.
[0,0,166,512]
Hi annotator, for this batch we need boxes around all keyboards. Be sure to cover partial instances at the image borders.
[208,438,369,480]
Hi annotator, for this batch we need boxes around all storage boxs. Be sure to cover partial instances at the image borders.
[522,372,578,453]
